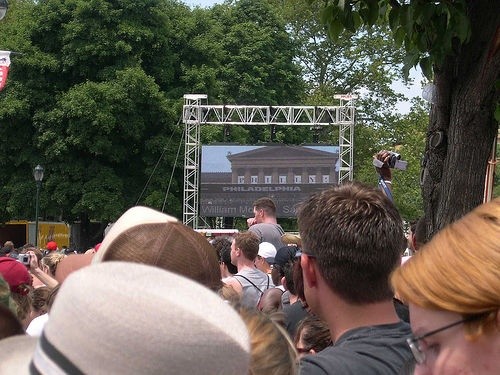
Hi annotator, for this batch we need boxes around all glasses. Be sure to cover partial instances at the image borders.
[406,316,477,365]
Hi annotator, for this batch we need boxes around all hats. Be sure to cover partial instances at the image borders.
[47,241,57,250]
[258,242,277,258]
[0,256,33,295]
[91,205,222,290]
[0,260,250,375]
[266,246,299,264]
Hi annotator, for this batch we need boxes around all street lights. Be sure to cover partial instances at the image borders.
[32,163,45,248]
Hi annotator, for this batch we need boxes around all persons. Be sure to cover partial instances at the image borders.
[374,149,426,322]
[0,206,251,375]
[299,185,418,375]
[212,197,332,375]
[391,197,500,375]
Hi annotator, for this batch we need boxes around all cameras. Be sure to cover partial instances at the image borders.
[19,254,31,265]
[386,150,407,170]
